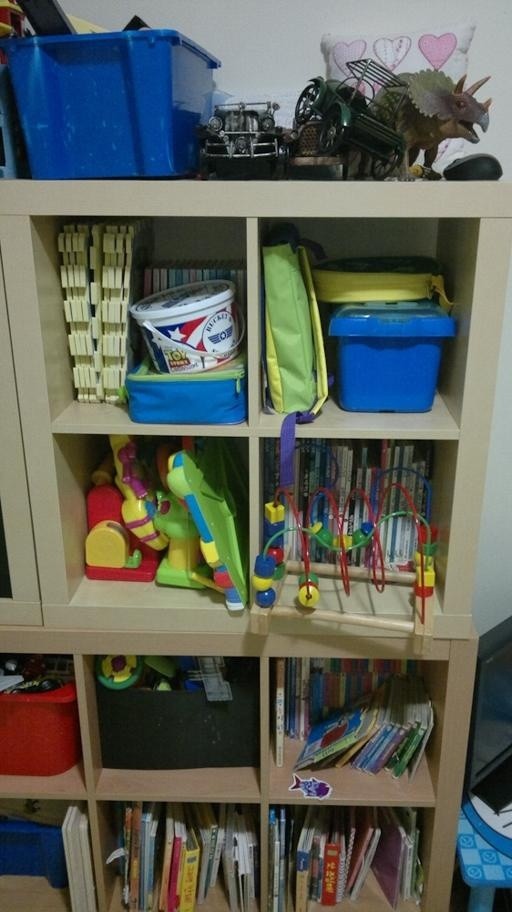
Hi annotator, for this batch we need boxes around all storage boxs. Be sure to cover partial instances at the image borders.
[0,823,65,887]
[0,30,221,180]
[95,681,260,770]
[0,684,75,774]
[327,300,459,413]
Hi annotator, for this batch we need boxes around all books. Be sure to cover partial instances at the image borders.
[62,800,97,912]
[106,801,260,912]
[265,440,426,567]
[269,808,424,912]
[276,658,433,782]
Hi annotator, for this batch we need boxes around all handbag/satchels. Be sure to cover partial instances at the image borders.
[313,255,443,303]
[260,238,328,423]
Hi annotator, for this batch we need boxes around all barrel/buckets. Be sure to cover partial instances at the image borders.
[128,276,245,375]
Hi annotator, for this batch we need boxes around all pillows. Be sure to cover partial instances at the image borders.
[320,24,477,171]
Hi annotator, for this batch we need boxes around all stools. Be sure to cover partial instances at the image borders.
[458,809,512,912]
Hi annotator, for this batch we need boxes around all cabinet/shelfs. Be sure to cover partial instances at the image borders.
[0,181,512,912]
